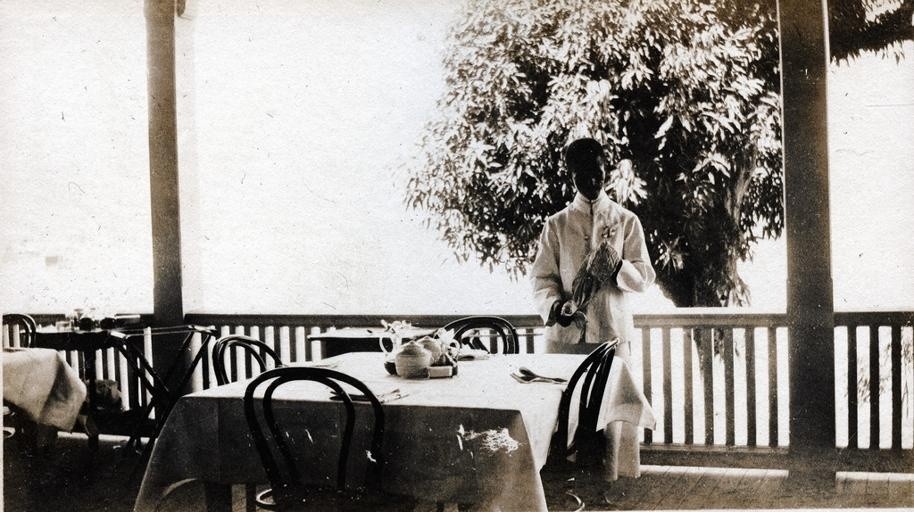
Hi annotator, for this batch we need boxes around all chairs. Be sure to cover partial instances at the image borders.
[4,312,37,468]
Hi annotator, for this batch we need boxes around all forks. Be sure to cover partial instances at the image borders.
[510,373,561,384]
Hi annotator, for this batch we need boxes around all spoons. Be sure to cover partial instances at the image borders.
[519,367,568,382]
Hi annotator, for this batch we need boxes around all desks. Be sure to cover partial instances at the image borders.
[181,351,619,512]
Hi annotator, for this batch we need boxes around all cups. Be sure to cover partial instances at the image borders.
[395,336,442,380]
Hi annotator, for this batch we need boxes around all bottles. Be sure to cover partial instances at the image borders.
[380,332,405,376]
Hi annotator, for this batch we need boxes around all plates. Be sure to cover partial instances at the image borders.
[330,379,402,397]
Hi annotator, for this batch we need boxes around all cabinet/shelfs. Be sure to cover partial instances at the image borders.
[20,320,148,458]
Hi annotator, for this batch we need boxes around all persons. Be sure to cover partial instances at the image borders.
[530,139,657,369]
